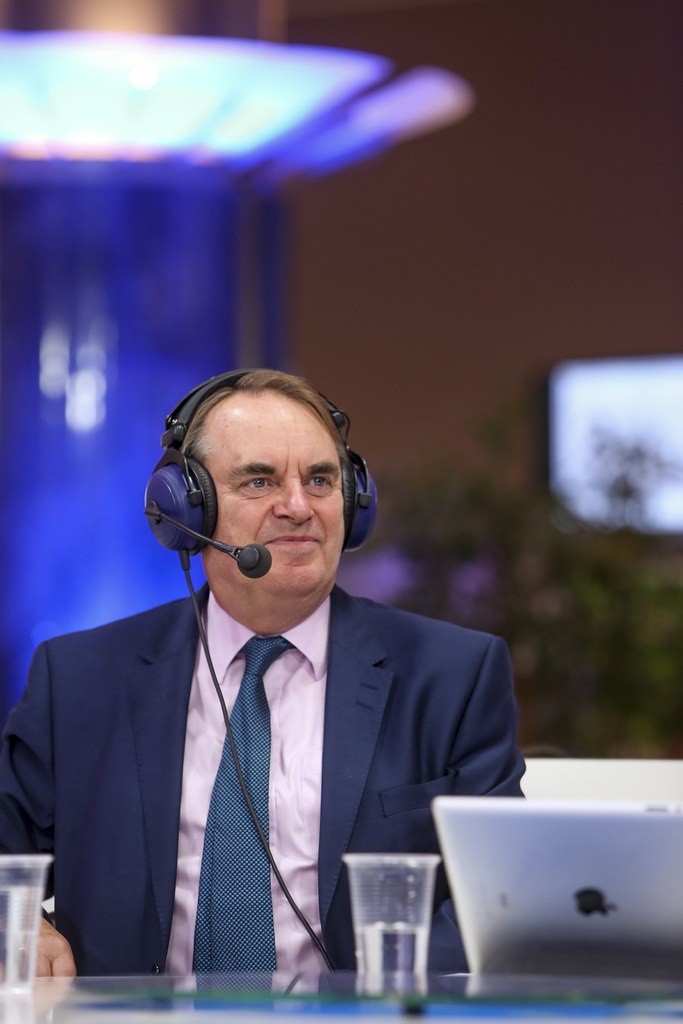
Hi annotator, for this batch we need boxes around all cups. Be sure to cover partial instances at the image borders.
[341,852,442,999]
[0,853,56,1023]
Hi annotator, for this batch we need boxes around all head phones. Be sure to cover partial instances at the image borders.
[142,368,378,570]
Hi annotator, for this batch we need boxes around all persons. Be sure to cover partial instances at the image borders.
[1,367,527,978]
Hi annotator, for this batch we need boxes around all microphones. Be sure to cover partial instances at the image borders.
[144,507,273,580]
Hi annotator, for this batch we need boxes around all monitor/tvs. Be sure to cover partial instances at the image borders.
[529,355,683,538]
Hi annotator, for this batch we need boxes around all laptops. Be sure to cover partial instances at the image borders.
[430,796,681,1002]
